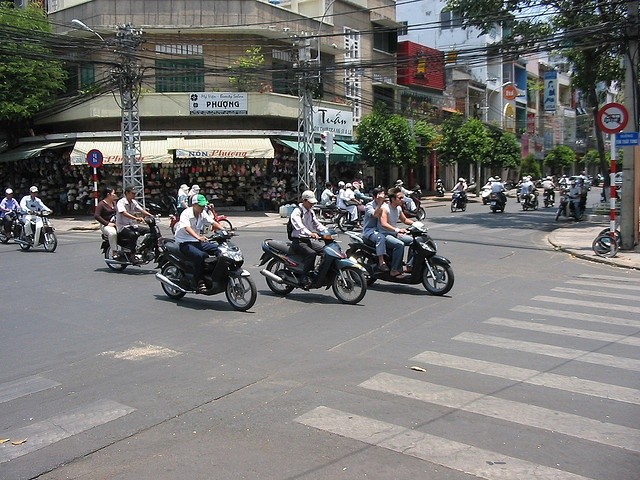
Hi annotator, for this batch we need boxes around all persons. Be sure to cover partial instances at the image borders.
[394,179,417,213]
[526,175,539,205]
[175,194,225,293]
[19,186,53,243]
[364,186,390,272]
[579,173,585,177]
[491,176,507,204]
[344,183,367,214]
[547,80,557,101]
[353,182,374,212]
[451,177,468,203]
[377,187,416,276]
[577,179,587,212]
[188,184,200,205]
[599,173,611,203]
[541,175,555,200]
[336,181,358,225]
[516,176,536,205]
[116,186,154,264]
[559,174,570,185]
[290,190,332,286]
[514,178,524,189]
[482,176,495,190]
[561,180,581,221]
[176,184,189,216]
[0,188,21,237]
[93,187,117,258]
[321,182,337,211]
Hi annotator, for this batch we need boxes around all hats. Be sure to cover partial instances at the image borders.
[493,176,502,181]
[30,186,38,193]
[192,185,200,190]
[1,155,93,185]
[523,177,530,182]
[6,188,13,195]
[546,176,552,179]
[405,190,414,195]
[144,162,178,203]
[94,165,123,196]
[40,187,94,214]
[458,178,463,182]
[302,190,318,204]
[395,180,403,186]
[527,175,532,179]
[192,194,209,207]
[125,184,138,194]
[179,153,296,184]
[571,182,575,184]
[201,187,293,206]
[180,184,190,190]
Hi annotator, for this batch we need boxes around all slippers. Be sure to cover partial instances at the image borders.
[391,272,405,280]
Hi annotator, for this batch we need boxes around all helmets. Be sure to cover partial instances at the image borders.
[575,178,584,187]
[346,183,352,189]
[338,181,345,187]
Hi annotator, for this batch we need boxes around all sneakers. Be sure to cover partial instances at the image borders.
[113,250,120,258]
[378,262,390,272]
[301,276,312,284]
[25,237,31,243]
[197,280,208,294]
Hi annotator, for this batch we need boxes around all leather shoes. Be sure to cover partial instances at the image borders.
[313,266,319,280]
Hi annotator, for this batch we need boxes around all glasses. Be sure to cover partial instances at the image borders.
[397,196,404,199]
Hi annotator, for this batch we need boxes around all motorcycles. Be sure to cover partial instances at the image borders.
[333,196,376,232]
[489,189,508,212]
[344,220,454,295]
[398,190,426,220]
[14,210,57,252]
[154,230,257,311]
[101,215,175,272]
[559,183,569,196]
[258,229,367,305]
[0,209,21,243]
[312,195,341,227]
[168,203,233,234]
[556,193,587,222]
[450,190,468,213]
[521,188,539,210]
[543,186,556,208]
[482,187,493,205]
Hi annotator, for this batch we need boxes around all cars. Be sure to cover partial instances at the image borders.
[566,176,590,192]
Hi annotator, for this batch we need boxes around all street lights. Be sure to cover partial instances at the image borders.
[71,18,110,51]
[484,81,512,185]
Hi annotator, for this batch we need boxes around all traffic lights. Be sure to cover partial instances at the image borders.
[320,133,327,151]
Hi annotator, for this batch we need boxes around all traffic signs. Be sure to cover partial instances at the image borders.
[616,132,638,147]
